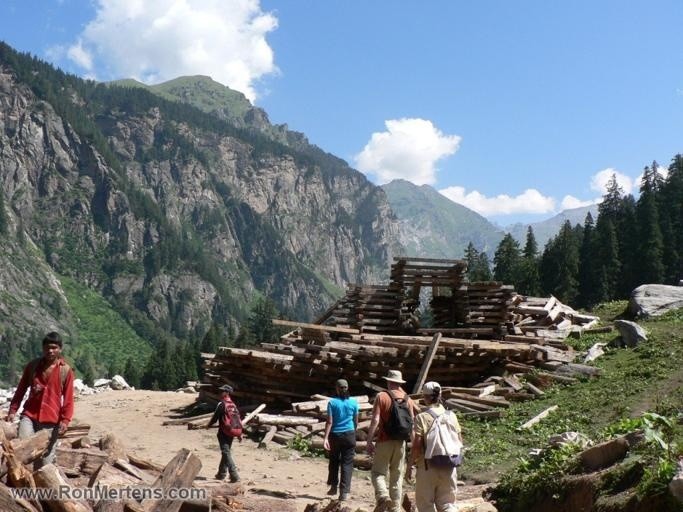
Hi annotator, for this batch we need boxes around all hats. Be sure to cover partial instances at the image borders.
[422,382,441,395]
[381,370,406,384]
[218,385,233,395]
[335,379,348,391]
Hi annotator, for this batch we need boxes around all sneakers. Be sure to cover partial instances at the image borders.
[327,488,336,495]
[227,479,240,483]
[215,475,225,480]
[338,494,347,501]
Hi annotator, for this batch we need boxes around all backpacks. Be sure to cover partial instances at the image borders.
[421,409,464,471]
[379,390,413,441]
[219,400,242,437]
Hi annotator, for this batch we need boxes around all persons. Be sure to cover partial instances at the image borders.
[206,384,243,483]
[367,369,415,512]
[6,332,74,473]
[405,381,461,512]
[323,379,359,501]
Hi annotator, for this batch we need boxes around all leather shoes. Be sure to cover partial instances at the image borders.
[373,497,392,512]
